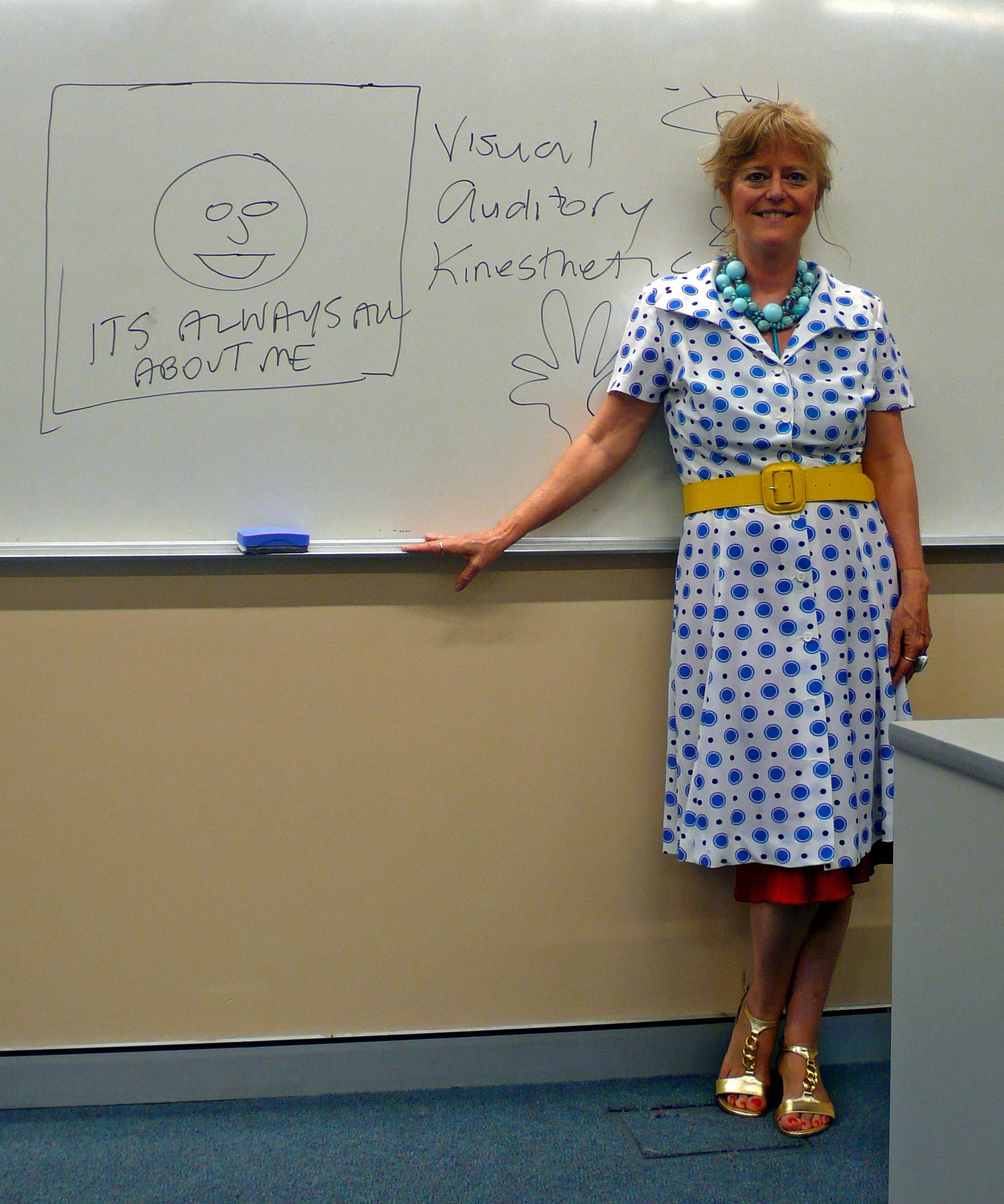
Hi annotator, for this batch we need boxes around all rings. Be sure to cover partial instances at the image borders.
[903,655,917,662]
[439,541,444,555]
[914,653,928,672]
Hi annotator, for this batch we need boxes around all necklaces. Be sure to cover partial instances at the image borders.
[716,250,815,357]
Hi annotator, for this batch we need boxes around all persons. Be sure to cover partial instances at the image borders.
[400,102,935,1135]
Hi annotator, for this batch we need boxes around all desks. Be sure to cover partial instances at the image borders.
[888,718,1004,1204]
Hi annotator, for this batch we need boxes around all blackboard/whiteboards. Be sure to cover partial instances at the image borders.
[0,0,1003,558]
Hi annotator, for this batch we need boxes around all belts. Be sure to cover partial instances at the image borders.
[682,462,878,515]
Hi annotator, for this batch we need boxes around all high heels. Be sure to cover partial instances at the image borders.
[715,985,779,1116]
[777,1041,836,1137]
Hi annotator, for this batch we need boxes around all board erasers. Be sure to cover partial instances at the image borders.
[236,526,311,555]
